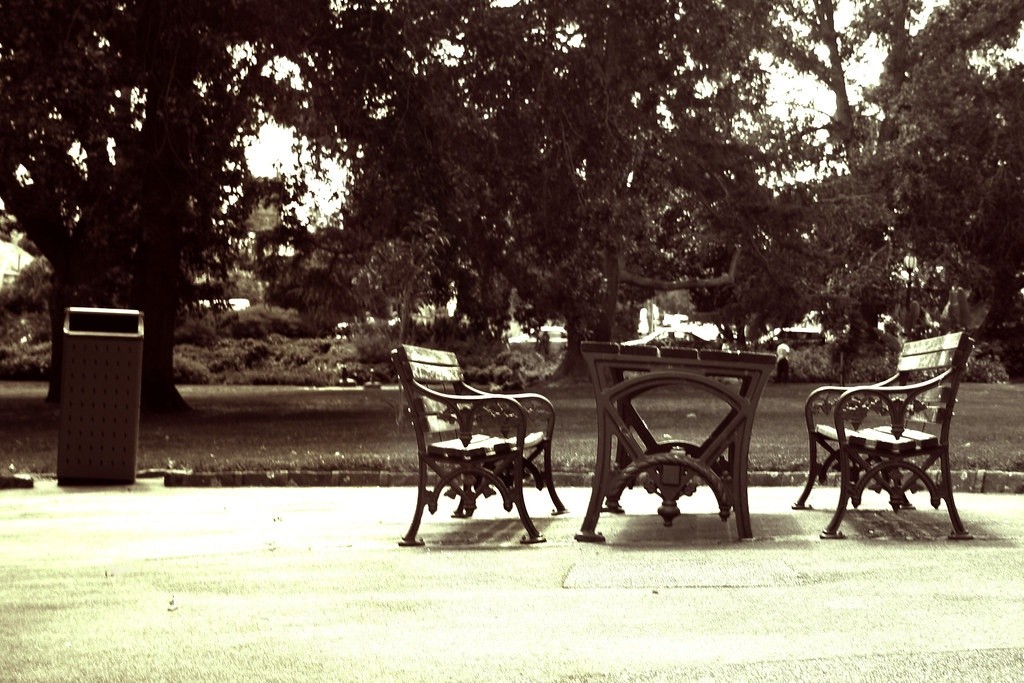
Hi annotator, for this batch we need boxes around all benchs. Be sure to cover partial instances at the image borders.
[390,343,570,546]
[792,331,976,541]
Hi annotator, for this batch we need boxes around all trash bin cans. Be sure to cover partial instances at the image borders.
[54,304,146,487]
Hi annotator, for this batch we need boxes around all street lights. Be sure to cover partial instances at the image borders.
[904,251,917,342]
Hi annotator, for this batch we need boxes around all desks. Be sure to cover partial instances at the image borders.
[574,341,777,544]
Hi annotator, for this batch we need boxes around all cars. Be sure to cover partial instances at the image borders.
[760,328,825,348]
[656,314,671,327]
[508,326,568,343]
[333,312,400,338]
[621,327,723,350]
